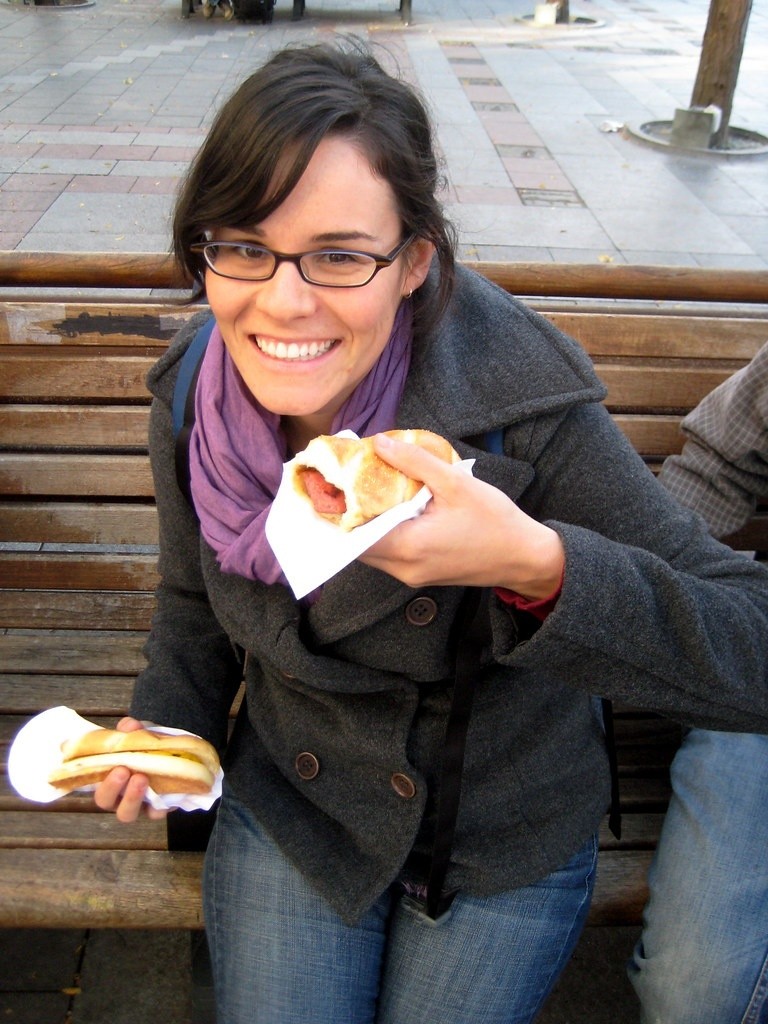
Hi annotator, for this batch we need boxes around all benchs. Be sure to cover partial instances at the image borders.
[0,249,768,991]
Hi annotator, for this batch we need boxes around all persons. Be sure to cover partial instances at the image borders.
[625,340,768,1024]
[92,44,768,1024]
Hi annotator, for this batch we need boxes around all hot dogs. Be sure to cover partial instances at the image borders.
[47,729,221,794]
[293,428,461,530]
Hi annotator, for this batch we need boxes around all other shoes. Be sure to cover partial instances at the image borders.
[203,0,216,18]
[217,0,234,20]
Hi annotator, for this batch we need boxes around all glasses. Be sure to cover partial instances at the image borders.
[202,230,416,288]
